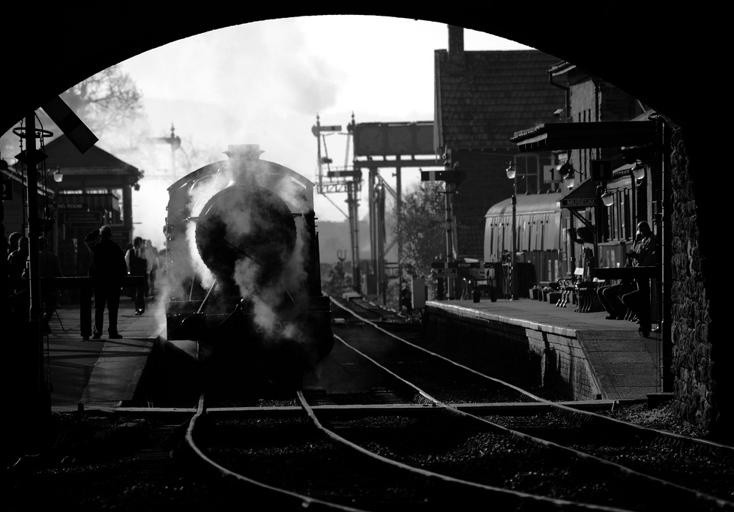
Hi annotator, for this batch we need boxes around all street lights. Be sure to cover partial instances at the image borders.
[556,162,583,274]
[505,161,525,300]
[52,166,64,256]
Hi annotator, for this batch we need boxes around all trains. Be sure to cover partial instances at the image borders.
[164,144,335,380]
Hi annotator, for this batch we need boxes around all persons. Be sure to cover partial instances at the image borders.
[597,277,627,320]
[0,227,156,335]
[628,221,655,289]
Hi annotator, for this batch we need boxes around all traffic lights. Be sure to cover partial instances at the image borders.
[421,171,448,180]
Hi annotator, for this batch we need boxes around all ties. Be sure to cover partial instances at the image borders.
[136,249,138,257]
